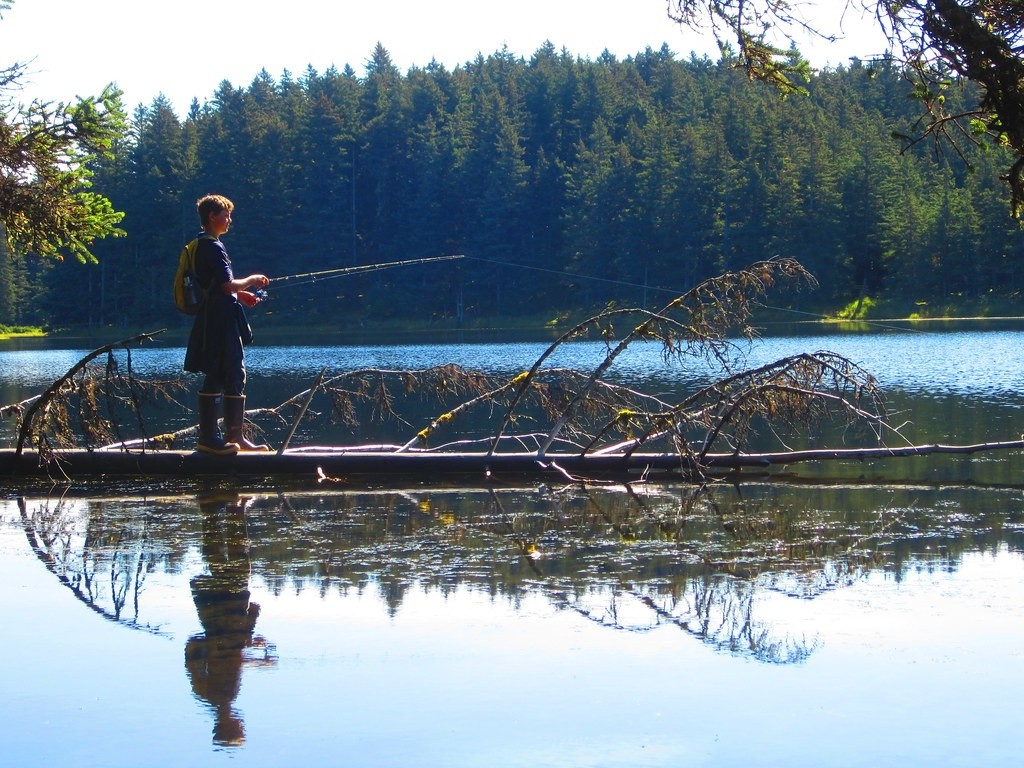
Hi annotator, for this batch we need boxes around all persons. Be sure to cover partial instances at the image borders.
[190,492,277,746]
[184,195,270,455]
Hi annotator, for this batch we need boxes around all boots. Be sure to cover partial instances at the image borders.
[223,394,269,451]
[196,390,237,456]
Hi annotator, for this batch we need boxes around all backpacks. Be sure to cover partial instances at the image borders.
[174,238,209,315]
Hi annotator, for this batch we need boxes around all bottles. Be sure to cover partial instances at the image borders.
[183,276,192,287]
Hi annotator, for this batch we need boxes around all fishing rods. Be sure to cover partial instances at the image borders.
[239,252,468,308]
